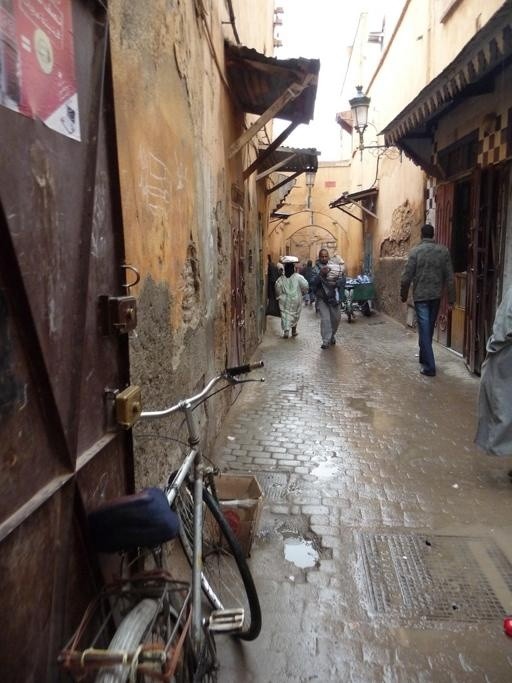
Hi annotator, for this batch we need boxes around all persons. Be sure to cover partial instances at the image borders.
[473,284,512,477]
[401,224,456,376]
[268,249,346,350]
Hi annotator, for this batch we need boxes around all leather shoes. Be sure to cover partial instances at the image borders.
[418,367,436,377]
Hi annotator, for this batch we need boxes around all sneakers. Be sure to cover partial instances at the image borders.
[320,337,337,350]
[282,330,300,339]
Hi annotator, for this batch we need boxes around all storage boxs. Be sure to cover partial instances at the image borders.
[175,468,265,563]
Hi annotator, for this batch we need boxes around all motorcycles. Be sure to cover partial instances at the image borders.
[340,284,361,323]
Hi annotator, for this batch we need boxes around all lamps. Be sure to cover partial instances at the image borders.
[345,81,404,166]
[306,160,318,208]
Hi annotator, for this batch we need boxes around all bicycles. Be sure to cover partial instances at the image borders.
[56,359,266,683]
